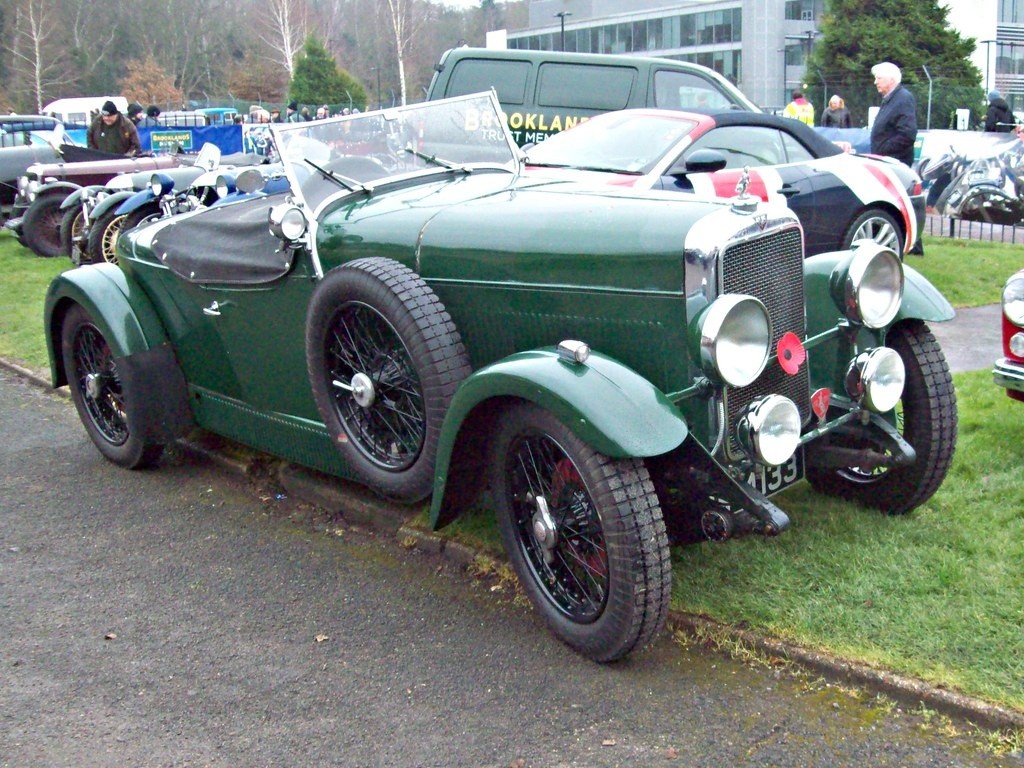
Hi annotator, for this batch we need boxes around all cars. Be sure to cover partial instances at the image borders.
[1,110,290,265]
[41,85,958,665]
[990,269,1024,403]
[504,108,927,264]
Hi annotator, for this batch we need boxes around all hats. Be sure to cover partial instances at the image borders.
[146,106,160,117]
[101,101,118,116]
[288,101,298,111]
[127,104,143,118]
[988,90,1001,103]
[270,107,281,113]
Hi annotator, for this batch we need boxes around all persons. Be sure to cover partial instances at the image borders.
[870,62,924,256]
[127,104,143,125]
[6,107,17,116]
[821,94,853,128]
[86,100,142,155]
[783,91,814,126]
[136,105,161,127]
[984,90,1016,133]
[90,108,100,122]
[233,101,360,124]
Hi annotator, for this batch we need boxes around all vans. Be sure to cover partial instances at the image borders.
[401,45,763,174]
